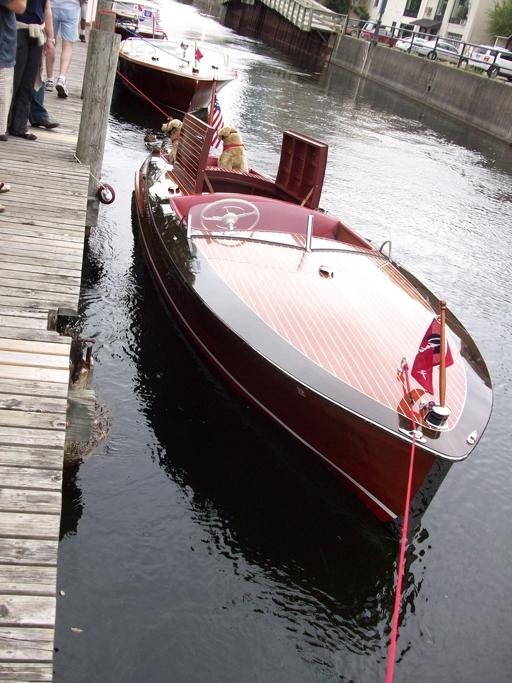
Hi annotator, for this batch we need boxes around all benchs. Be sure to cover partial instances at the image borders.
[172,191,364,247]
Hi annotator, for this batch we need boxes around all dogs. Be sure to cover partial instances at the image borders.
[215,124,249,173]
[161,117,184,162]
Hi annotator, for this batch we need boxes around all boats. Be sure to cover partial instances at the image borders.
[117,35,238,121]
[113,0,167,42]
[135,112,497,527]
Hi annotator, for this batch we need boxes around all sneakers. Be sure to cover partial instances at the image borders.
[5,127,37,140]
[45,79,53,92]
[31,118,59,129]
[56,80,68,98]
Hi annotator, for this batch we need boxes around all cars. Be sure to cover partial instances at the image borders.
[339,18,469,67]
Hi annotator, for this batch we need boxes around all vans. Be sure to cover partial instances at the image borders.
[466,43,512,82]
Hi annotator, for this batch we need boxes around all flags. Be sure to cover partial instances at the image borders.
[208,92,225,150]
[411,318,453,395]
[153,15,168,32]
[195,47,204,62]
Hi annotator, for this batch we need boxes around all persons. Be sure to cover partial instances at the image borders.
[0,0,88,141]
